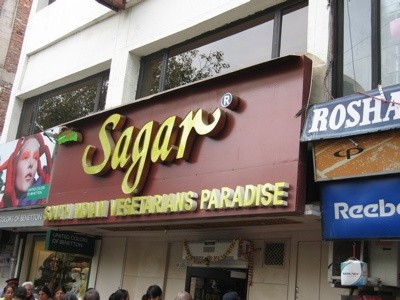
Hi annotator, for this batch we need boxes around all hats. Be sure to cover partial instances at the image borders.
[5,277,19,286]
[222,292,240,300]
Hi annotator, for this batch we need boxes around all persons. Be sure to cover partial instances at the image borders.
[0,277,242,300]
[2,138,45,206]
